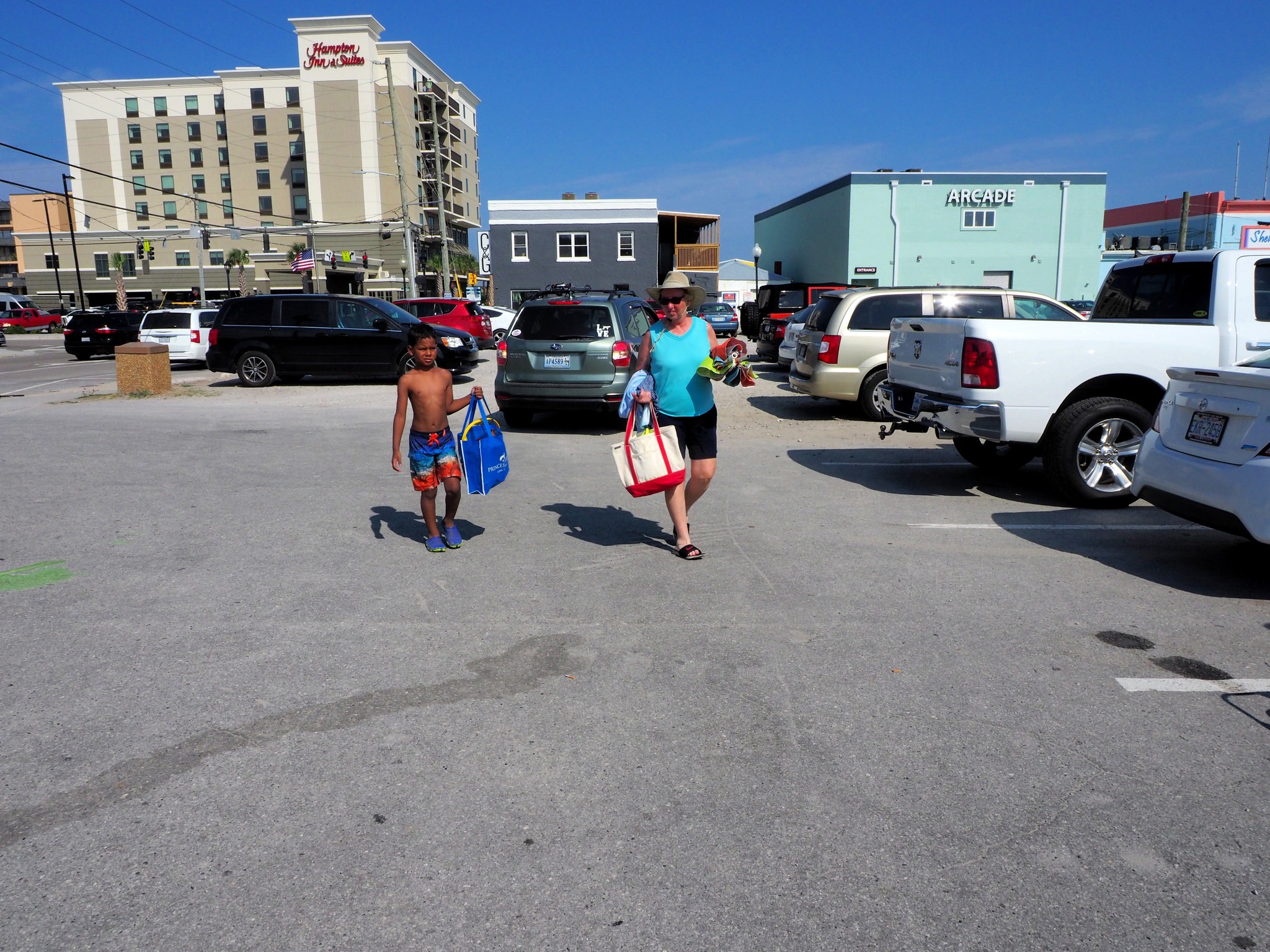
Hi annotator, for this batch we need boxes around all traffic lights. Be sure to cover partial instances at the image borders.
[148,246,155,261]
[137,245,144,259]
[191,287,202,297]
[203,233,210,249]
[362,255,369,269]
[414,238,422,253]
[331,257,337,270]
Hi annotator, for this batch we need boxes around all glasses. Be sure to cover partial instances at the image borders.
[658,294,686,305]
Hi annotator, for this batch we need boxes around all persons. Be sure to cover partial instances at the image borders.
[391,322,483,552]
[632,271,719,559]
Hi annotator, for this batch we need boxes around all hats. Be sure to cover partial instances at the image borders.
[646,271,707,311]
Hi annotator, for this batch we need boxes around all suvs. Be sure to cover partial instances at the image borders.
[205,293,479,389]
[63,310,146,361]
[137,305,220,364]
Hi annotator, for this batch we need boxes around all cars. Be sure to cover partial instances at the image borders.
[687,303,739,338]
[1131,346,1270,546]
[0,292,225,345]
[738,281,1095,423]
[493,283,667,428]
[367,297,519,350]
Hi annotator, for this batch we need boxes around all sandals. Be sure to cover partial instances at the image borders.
[426,537,446,552]
[441,522,462,548]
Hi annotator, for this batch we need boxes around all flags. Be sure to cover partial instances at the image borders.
[291,249,315,272]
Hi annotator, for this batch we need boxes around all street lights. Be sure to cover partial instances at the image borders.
[433,267,438,295]
[224,259,232,298]
[352,171,418,318]
[419,255,428,296]
[32,198,65,313]
[752,243,764,303]
[399,255,409,298]
[61,173,87,314]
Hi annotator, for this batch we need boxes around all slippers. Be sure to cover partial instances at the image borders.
[672,544,705,559]
[673,522,690,541]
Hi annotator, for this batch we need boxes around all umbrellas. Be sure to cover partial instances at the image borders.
[696,337,759,388]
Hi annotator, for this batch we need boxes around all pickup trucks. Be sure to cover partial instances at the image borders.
[876,249,1270,511]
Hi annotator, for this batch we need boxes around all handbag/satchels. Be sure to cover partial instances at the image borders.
[611,397,686,498]
[457,390,509,496]
